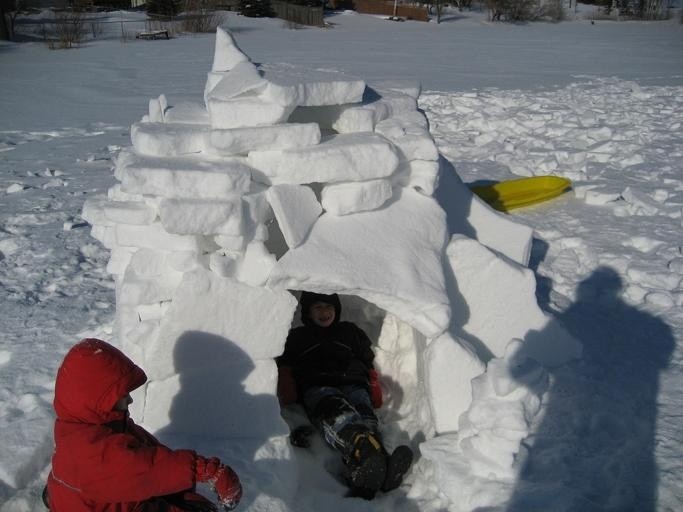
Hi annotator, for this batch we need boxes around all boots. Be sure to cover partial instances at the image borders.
[346,435,413,499]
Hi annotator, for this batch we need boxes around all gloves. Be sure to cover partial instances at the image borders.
[290,426,314,448]
[369,370,383,408]
[203,456,242,510]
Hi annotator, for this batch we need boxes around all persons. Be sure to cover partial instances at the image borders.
[510,260,676,505]
[274,291,414,500]
[44,337,244,512]
